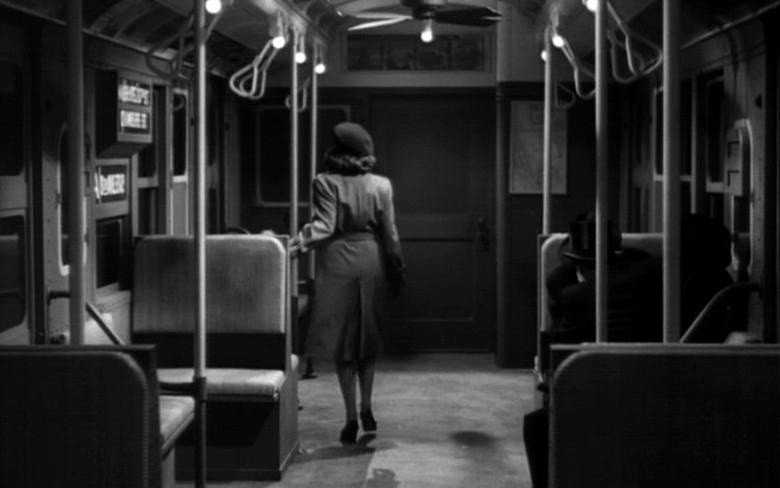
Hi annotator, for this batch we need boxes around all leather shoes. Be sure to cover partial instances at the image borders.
[339,420,359,444]
[360,404,378,432]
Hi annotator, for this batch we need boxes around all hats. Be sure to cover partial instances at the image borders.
[334,121,374,159]
[562,214,625,262]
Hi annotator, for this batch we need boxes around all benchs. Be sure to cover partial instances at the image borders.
[0,344,160,488]
[129,235,301,481]
[159,394,195,488]
[549,343,780,488]
[533,232,664,409]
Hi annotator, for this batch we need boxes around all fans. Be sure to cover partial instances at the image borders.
[346,1,503,31]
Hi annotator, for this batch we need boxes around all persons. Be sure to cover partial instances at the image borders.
[260,119,405,444]
[546,236,586,320]
[662,214,744,331]
[545,210,655,328]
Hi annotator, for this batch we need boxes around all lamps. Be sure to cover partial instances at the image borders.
[541,0,599,62]
[202,0,326,75]
[413,10,437,43]
